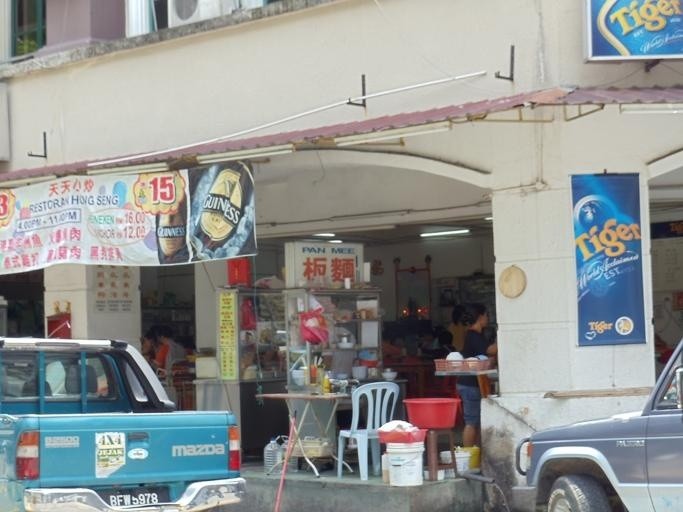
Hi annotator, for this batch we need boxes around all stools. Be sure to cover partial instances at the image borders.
[423,427,458,478]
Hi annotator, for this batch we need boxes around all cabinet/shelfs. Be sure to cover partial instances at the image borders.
[193,288,408,469]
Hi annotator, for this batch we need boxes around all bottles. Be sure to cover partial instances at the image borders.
[155,191,189,264]
[380,450,390,485]
[191,157,255,259]
[291,364,359,395]
[263,437,298,474]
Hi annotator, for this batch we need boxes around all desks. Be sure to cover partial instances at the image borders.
[255,393,354,478]
[434,368,498,397]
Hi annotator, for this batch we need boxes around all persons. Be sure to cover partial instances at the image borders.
[453,304,498,449]
[139,325,196,379]
[380,303,496,367]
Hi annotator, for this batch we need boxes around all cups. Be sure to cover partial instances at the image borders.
[344,278,352,289]
[339,334,350,342]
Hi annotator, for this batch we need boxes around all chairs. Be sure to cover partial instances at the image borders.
[22,381,49,398]
[62,365,98,397]
[336,380,400,480]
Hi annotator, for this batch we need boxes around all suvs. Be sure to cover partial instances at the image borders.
[514,339,681,510]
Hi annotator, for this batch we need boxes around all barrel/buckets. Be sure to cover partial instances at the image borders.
[282,439,298,473]
[454,446,481,475]
[262,439,282,474]
[384,442,429,487]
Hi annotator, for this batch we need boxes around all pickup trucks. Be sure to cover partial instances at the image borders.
[0,337,246,511]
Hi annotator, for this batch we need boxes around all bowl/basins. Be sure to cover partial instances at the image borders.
[351,362,399,380]
[424,470,446,481]
[337,342,355,349]
[402,396,461,428]
[445,350,488,371]
